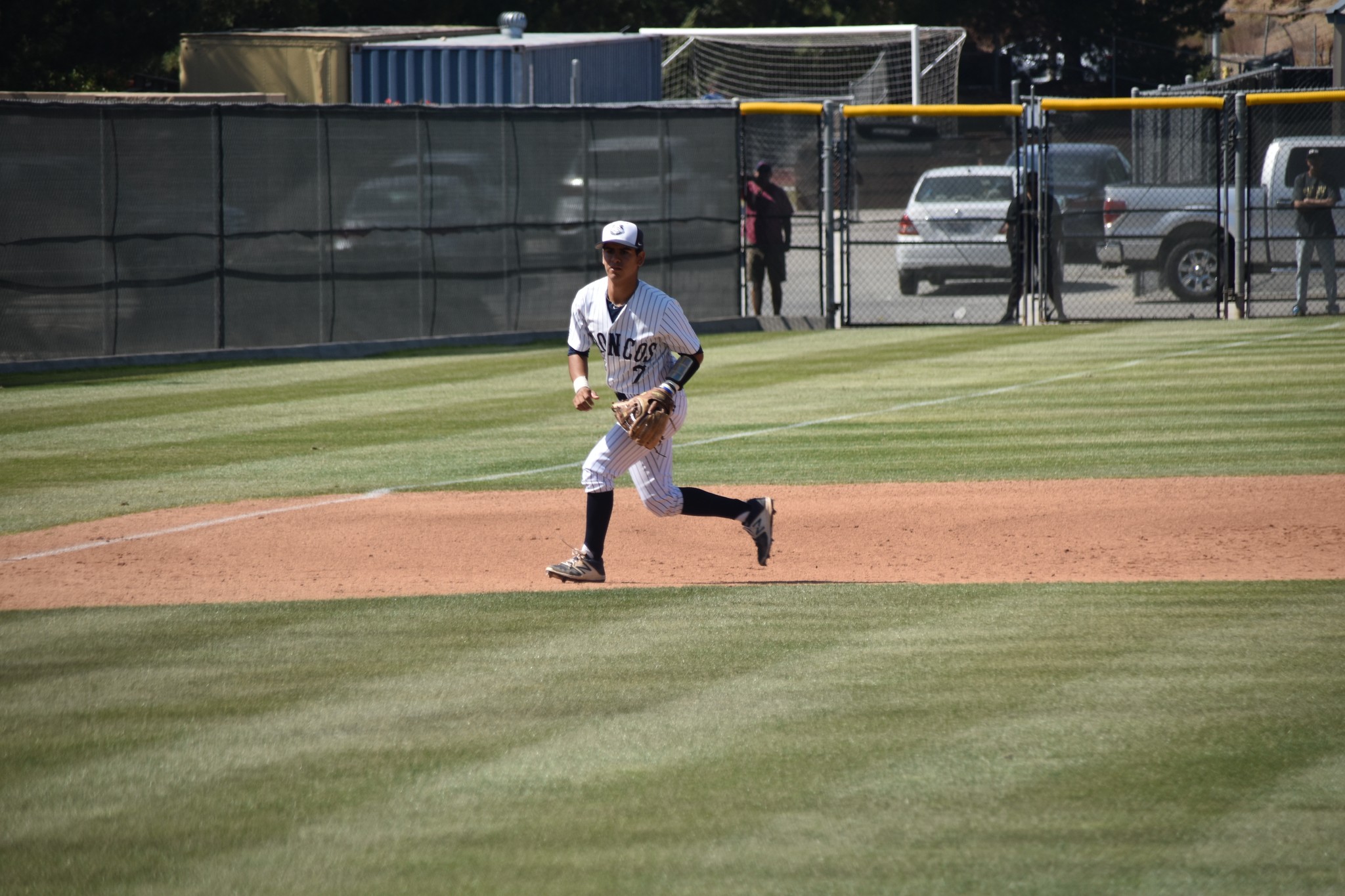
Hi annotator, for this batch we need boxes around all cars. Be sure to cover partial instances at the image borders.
[894,164,1064,295]
[329,147,518,261]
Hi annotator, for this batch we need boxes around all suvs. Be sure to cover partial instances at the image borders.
[548,134,712,264]
[1007,142,1133,263]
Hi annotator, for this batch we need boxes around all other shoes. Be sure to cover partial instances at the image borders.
[1329,306,1340,315]
[1000,314,1014,324]
[1059,315,1066,320]
[1293,306,1307,316]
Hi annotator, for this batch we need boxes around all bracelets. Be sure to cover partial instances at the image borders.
[659,383,675,397]
[573,376,590,393]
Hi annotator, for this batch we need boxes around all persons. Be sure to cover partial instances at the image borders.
[544,221,777,583]
[996,171,1071,326]
[1289,148,1340,316]
[740,161,794,318]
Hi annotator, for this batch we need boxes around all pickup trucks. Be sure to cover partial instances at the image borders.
[1095,135,1345,304]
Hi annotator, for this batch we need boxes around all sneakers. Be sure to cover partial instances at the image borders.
[545,546,607,583]
[742,495,776,566]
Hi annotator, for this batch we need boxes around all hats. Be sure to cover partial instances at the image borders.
[754,161,772,173]
[1306,147,1321,157]
[595,220,644,252]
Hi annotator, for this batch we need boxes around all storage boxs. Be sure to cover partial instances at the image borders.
[350,31,661,105]
[179,24,499,105]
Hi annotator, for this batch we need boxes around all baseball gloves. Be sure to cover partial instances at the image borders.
[611,385,676,450]
